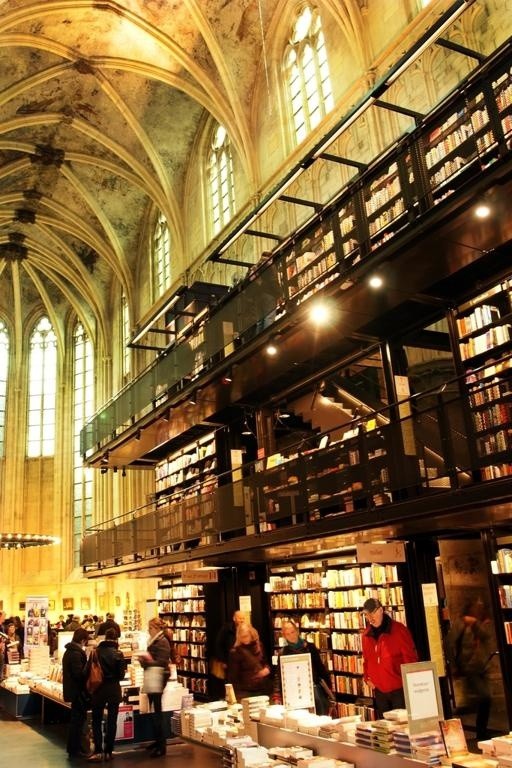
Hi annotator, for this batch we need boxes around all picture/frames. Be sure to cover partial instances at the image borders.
[0,592,121,612]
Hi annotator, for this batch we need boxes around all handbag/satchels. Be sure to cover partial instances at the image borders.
[142,666,165,694]
[85,650,105,695]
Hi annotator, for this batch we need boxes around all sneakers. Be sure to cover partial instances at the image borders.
[146,742,167,759]
[68,754,94,762]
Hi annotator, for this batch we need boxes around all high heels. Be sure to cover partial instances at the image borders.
[104,749,114,760]
[93,750,103,760]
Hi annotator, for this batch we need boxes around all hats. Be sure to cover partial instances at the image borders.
[72,615,80,622]
[359,598,382,615]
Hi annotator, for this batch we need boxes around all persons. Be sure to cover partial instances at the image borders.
[62,627,90,758]
[124,712,132,722]
[80,627,125,763]
[27,607,47,644]
[0,610,120,672]
[134,619,172,759]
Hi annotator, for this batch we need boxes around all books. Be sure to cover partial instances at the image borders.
[154,62,512,766]
[2,645,146,705]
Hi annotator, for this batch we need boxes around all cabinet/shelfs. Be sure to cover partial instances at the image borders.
[0,667,149,753]
